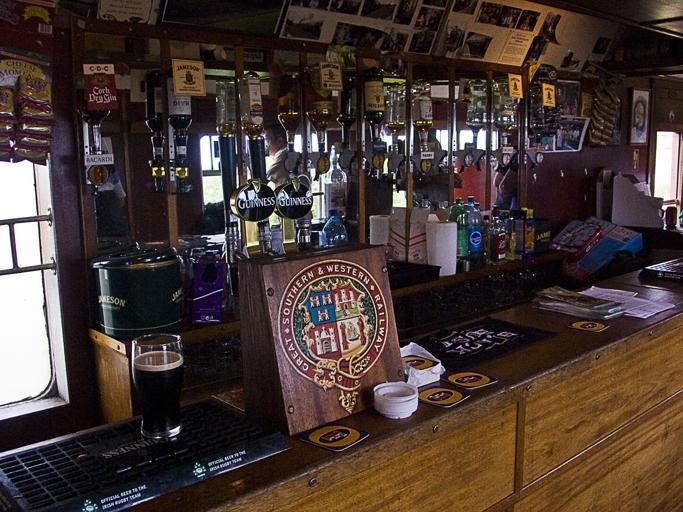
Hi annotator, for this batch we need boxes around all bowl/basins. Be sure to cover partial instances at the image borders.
[372,381,419,419]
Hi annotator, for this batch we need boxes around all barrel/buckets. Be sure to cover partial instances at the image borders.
[91,242,182,339]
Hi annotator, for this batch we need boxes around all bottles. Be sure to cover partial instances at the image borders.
[255,218,312,260]
[221,222,250,316]
[319,207,349,247]
[451,196,517,265]
[79,55,557,188]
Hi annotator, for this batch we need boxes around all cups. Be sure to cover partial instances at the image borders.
[130,333,186,443]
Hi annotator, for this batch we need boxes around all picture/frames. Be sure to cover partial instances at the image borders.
[629,86,653,148]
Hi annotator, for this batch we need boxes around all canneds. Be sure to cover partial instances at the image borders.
[666,207,677,226]
